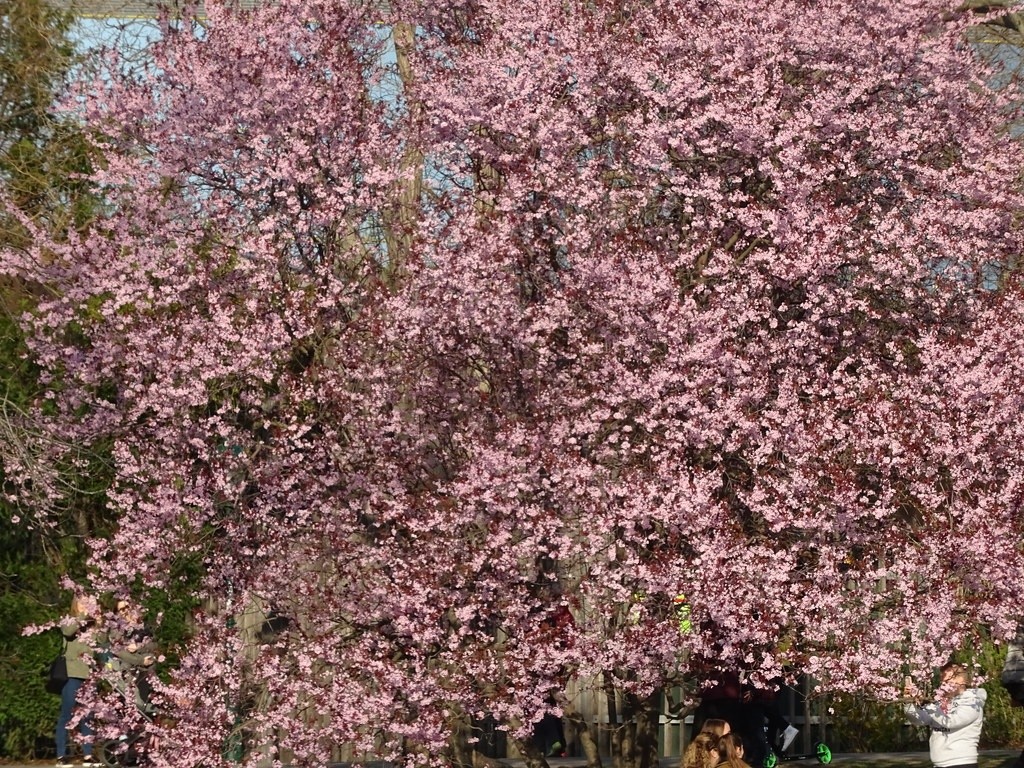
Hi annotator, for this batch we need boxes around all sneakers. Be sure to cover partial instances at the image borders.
[779,725,799,752]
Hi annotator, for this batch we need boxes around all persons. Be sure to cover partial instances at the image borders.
[54,592,163,768]
[902,663,987,768]
[680,719,752,768]
[743,676,799,751]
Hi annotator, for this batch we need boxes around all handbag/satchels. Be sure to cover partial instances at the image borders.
[45,635,69,694]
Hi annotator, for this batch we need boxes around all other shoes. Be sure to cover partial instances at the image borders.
[544,736,562,757]
[82,756,106,767]
[55,756,74,768]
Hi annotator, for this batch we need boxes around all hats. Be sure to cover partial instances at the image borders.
[812,738,823,746]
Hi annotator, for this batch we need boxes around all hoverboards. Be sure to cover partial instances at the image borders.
[761,741,833,768]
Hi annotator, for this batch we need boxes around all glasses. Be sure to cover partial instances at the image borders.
[118,604,130,614]
